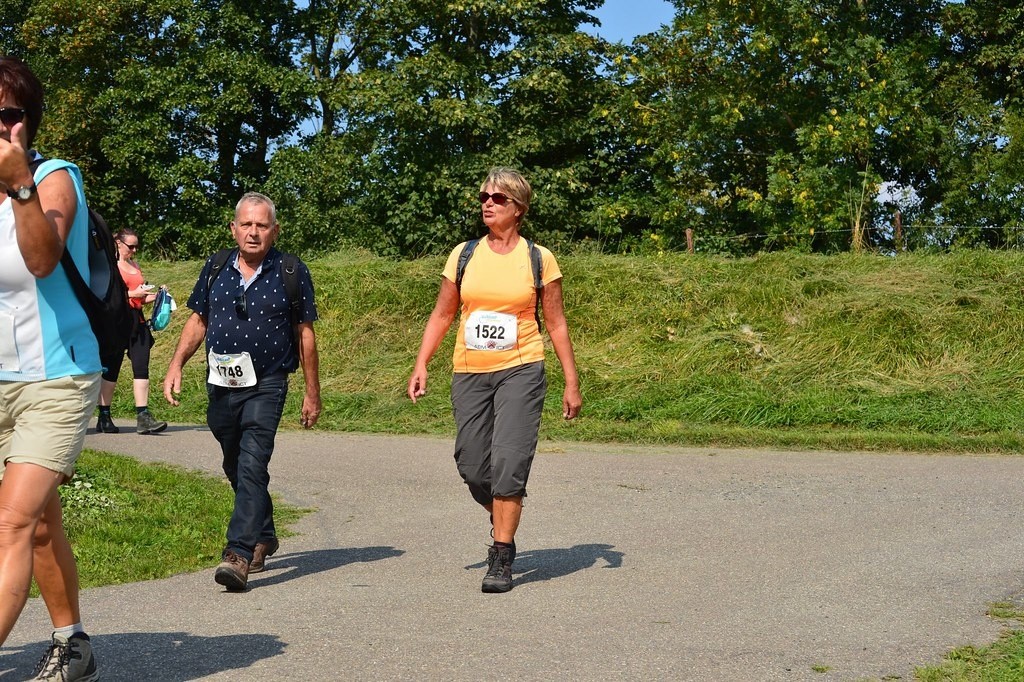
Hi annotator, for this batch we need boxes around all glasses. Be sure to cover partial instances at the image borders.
[479,192,513,205]
[118,238,138,249]
[0,107,26,125]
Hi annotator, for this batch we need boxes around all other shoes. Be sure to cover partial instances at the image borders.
[137,409,167,433]
[96,411,119,433]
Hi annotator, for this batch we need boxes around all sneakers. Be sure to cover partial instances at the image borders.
[482,544,516,591]
[249,540,279,572]
[23,632,100,682]
[215,549,250,590]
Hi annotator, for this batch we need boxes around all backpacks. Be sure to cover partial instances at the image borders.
[28,159,133,358]
[146,286,171,331]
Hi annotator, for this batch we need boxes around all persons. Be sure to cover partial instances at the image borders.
[163,192,321,592]
[407,167,582,593]
[0,54,103,682]
[97,227,167,434]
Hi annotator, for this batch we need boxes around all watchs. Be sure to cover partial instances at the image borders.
[6,182,37,201]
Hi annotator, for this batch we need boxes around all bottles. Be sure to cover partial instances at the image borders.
[141,281,148,290]
[150,326,153,334]
